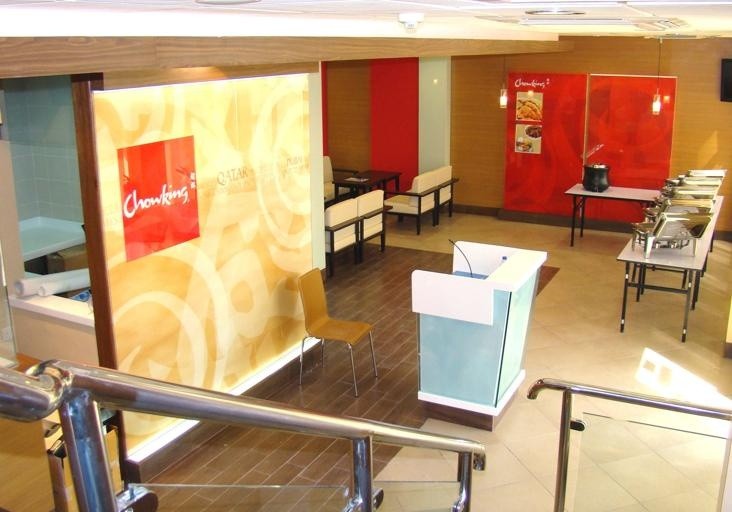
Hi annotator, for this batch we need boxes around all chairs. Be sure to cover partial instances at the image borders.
[323,167,359,209]
[381,177,460,236]
[296,267,379,398]
[324,203,393,276]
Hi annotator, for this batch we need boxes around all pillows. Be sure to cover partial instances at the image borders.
[323,156,334,183]
[325,189,385,244]
[409,165,452,207]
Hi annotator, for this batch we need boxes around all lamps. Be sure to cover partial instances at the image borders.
[651,39,663,116]
[499,55,508,109]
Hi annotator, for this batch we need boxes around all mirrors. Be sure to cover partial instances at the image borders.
[1,77,90,274]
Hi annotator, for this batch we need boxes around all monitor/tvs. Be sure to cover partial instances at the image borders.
[720,59,732,102]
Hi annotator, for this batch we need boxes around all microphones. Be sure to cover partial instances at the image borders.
[448,239,473,277]
